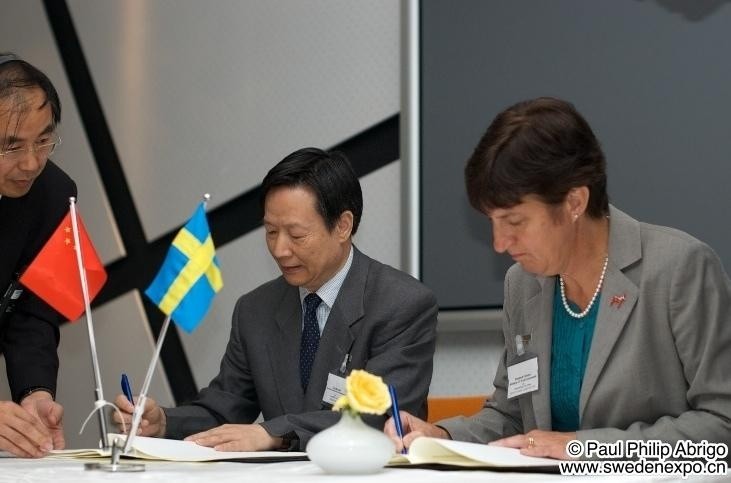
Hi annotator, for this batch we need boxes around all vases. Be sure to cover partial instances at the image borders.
[305,410,396,476]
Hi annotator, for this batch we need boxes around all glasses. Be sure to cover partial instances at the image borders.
[0,131,63,161]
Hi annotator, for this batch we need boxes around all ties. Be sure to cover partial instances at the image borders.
[299,292,323,396]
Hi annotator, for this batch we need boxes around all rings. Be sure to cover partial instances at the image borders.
[527,433,533,447]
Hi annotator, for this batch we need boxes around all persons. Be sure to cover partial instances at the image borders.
[383,96,731,467]
[112,146,440,453]
[1,47,78,459]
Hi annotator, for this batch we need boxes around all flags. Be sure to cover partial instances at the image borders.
[18,209,108,323]
[143,201,225,336]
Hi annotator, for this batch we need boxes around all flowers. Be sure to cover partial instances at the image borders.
[332,367,392,419]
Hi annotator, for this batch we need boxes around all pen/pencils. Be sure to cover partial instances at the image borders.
[121,374,134,407]
[387,383,407,455]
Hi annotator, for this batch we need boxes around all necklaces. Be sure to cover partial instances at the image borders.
[559,255,609,319]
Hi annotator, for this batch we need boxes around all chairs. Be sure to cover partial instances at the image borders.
[426,394,490,425]
